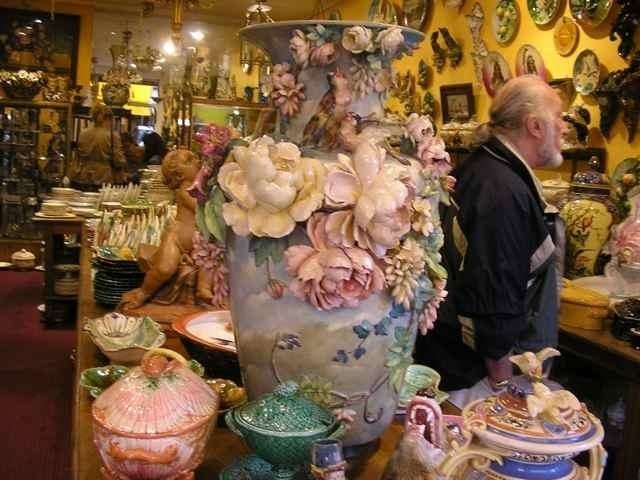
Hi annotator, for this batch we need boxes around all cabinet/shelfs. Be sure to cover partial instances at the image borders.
[0,108,70,239]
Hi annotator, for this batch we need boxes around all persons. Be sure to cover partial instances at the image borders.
[116,149,214,309]
[412,75,570,413]
[119,132,144,188]
[66,102,127,192]
[138,130,167,167]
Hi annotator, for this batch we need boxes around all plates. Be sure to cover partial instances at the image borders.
[76,358,249,415]
[550,15,580,59]
[81,311,168,365]
[35,212,77,218]
[70,191,103,218]
[571,48,603,94]
[51,277,80,296]
[567,0,613,29]
[171,308,238,357]
[527,1,564,28]
[88,244,148,309]
[492,0,522,47]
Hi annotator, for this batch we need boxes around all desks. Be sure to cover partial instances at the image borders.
[73,221,247,477]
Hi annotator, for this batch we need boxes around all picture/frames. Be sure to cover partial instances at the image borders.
[440,83,474,124]
[0,0,96,107]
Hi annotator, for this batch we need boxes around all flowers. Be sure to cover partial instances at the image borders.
[0,71,45,101]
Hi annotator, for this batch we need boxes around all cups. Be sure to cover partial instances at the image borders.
[41,201,74,216]
[52,187,84,201]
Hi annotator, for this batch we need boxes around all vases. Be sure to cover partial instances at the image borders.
[6,86,40,100]
[186,46,211,99]
[102,43,132,107]
[219,19,456,463]
